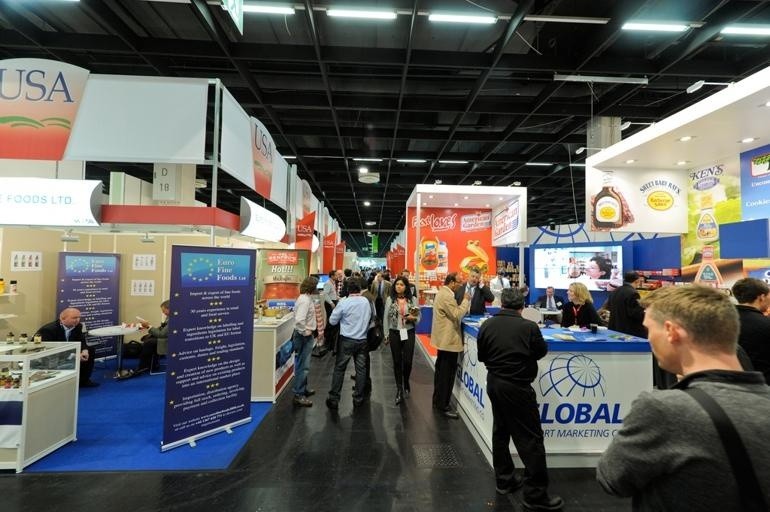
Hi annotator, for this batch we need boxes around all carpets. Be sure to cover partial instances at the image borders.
[22,354,274,474]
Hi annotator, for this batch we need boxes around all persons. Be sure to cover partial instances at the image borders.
[477,288,565,510]
[584,255,623,292]
[592,297,611,327]
[288,266,419,411]
[560,282,602,327]
[429,271,473,419]
[520,284,530,309]
[605,269,658,388]
[568,256,582,278]
[731,277,769,386]
[595,285,770,512]
[454,265,494,316]
[31,307,101,389]
[134,300,172,376]
[489,267,512,306]
[533,286,567,310]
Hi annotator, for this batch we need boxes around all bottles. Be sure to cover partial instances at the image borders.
[438,240,449,273]
[421,226,439,271]
[9,280,18,292]
[0,366,20,388]
[6,332,41,344]
[0,278,5,294]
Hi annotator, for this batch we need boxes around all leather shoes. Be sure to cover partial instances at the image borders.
[304,389,315,396]
[439,410,459,419]
[350,373,371,406]
[294,396,313,407]
[432,404,452,411]
[495,474,524,495]
[522,495,564,510]
[79,379,99,387]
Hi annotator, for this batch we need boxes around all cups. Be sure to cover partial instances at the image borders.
[591,325,597,335]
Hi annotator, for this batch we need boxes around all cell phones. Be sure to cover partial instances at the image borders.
[404,313,409,317]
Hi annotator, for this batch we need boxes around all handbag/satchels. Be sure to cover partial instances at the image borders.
[368,327,383,351]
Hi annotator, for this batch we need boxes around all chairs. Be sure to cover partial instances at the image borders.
[80,322,106,369]
[149,337,168,376]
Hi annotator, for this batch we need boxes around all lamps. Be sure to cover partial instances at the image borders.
[618,120,653,130]
[575,146,603,155]
[395,157,428,163]
[220,1,296,15]
[620,22,690,32]
[358,172,380,184]
[472,180,482,186]
[686,79,731,95]
[720,22,770,35]
[508,181,521,187]
[438,158,470,164]
[434,179,442,185]
[351,157,384,163]
[525,161,554,166]
[428,10,499,25]
[325,9,397,19]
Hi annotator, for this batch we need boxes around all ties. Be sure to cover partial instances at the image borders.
[549,297,552,308]
[377,282,381,297]
[466,287,471,295]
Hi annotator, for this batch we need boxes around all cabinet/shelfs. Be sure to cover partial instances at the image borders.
[0,292,20,320]
[250,310,295,405]
[0,341,82,474]
[408,271,526,294]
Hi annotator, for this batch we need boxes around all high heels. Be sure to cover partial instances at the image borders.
[395,387,403,405]
[404,380,410,394]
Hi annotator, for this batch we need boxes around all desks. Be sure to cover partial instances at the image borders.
[88,324,152,372]
